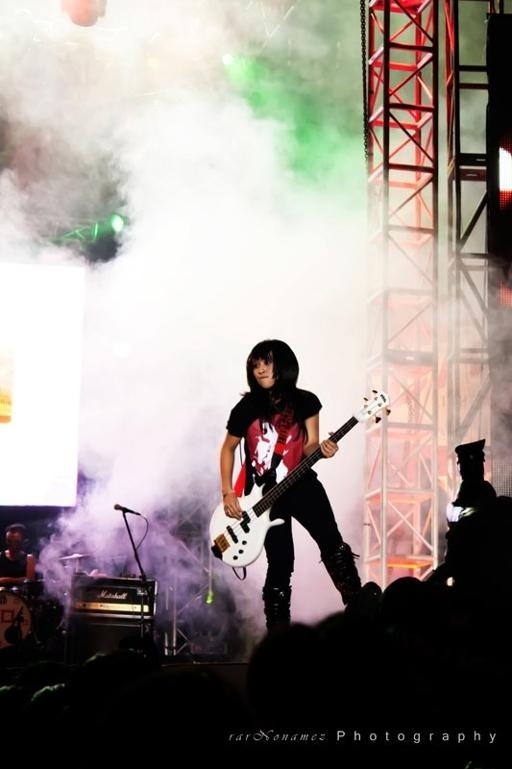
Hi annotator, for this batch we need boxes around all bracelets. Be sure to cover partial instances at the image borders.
[222,488,235,497]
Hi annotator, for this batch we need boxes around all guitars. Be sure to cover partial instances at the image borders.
[209,389,390,568]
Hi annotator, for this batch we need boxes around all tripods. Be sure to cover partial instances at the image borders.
[120,517,154,665]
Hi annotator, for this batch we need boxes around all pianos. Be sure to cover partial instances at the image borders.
[68,574,158,648]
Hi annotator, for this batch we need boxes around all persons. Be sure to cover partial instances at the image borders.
[1,608,512,768]
[218,339,363,635]
[0,522,36,595]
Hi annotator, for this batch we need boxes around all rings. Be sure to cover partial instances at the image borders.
[225,509,231,514]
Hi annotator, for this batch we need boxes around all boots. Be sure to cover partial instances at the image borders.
[324,545,361,604]
[263,586,292,632]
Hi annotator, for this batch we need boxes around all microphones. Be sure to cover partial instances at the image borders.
[114,503,141,517]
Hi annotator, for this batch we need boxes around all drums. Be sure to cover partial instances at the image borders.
[1,588,30,650]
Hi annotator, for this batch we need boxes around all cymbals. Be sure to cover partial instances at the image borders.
[58,555,89,559]
